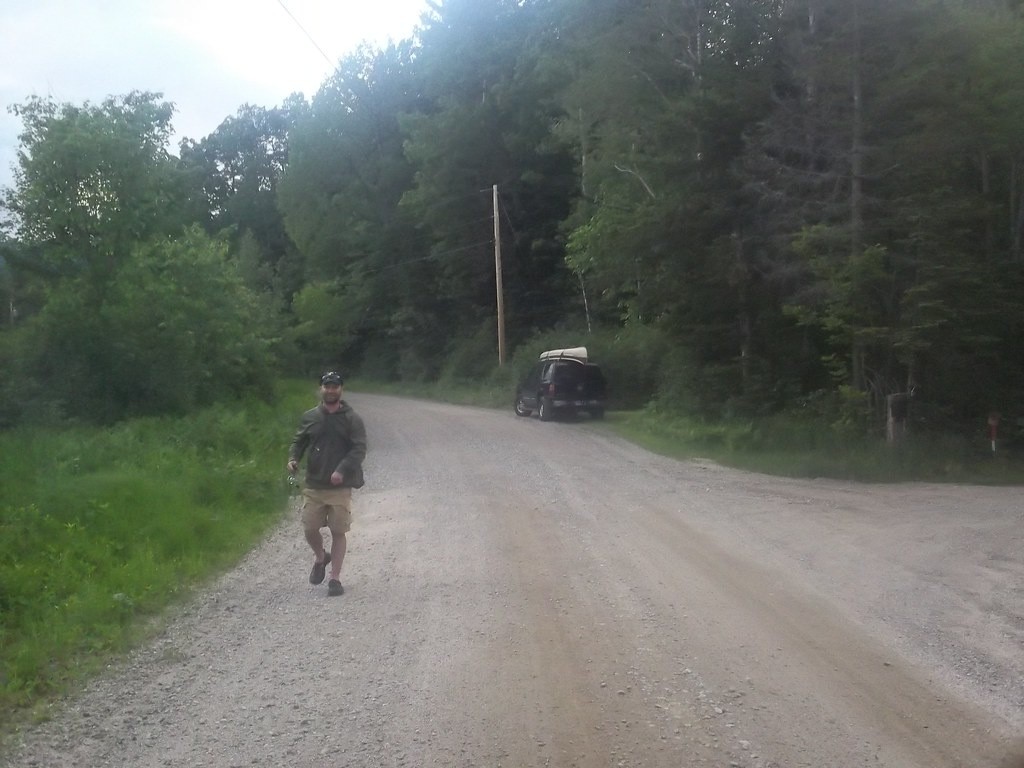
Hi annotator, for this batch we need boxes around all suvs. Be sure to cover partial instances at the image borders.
[514,346,608,421]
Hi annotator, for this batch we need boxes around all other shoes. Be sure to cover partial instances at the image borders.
[309,549,331,584]
[328,579,344,596]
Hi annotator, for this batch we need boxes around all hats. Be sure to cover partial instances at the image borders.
[320,372,344,385]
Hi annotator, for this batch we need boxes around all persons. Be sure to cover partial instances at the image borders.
[286,372,366,595]
